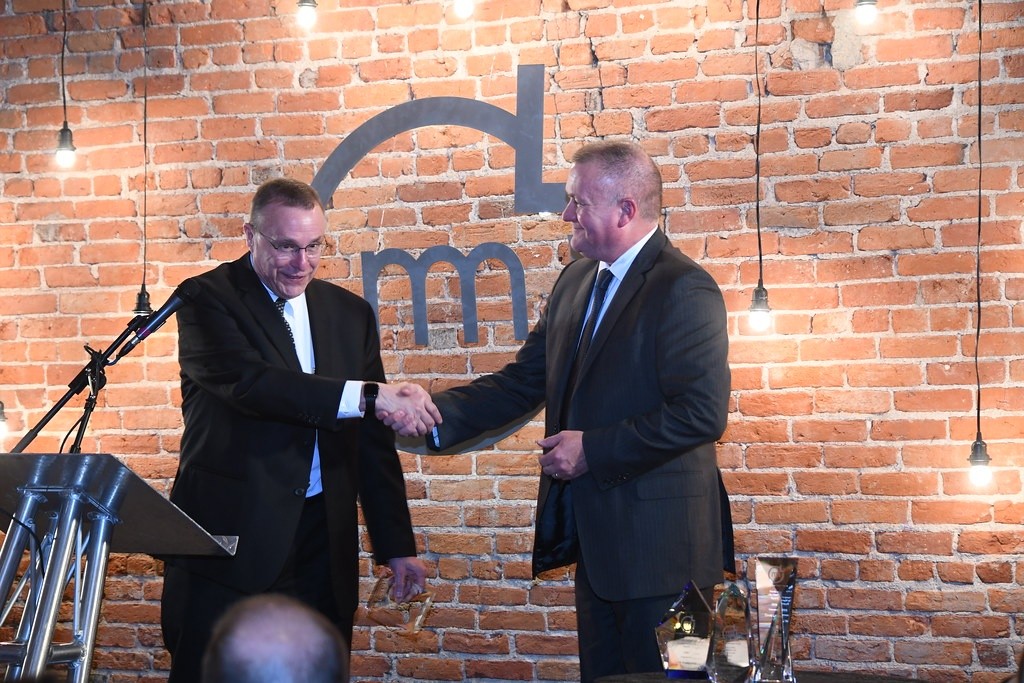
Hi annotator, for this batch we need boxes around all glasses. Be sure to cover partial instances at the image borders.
[259,229,329,258]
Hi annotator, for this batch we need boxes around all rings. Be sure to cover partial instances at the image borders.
[555,472,560,479]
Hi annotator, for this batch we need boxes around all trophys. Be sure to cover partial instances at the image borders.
[711,582,753,682]
[654,579,718,681]
[748,553,801,683]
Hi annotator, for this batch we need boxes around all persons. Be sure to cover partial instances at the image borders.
[158,178,430,683]
[202,594,352,683]
[377,142,738,683]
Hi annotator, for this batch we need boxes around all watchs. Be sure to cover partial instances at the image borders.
[362,380,380,419]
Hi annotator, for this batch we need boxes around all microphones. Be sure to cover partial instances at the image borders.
[115,278,201,361]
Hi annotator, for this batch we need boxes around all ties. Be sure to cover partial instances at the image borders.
[275,298,298,356]
[562,269,612,432]
